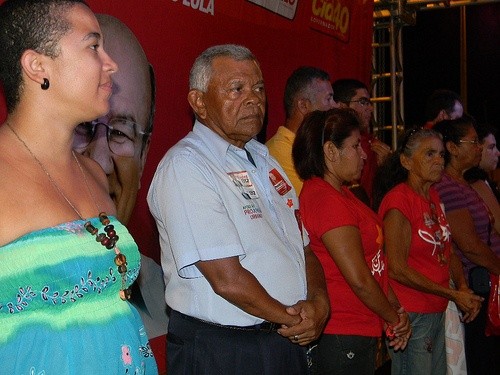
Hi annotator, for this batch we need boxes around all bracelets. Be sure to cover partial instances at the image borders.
[390,301,401,309]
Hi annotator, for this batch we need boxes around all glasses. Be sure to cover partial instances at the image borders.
[71,117,151,158]
[343,96,373,107]
[459,136,480,144]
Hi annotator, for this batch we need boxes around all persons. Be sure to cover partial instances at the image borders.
[463,124,500,236]
[331,78,395,210]
[376,129,485,375]
[421,98,464,130]
[73,13,170,340]
[292,107,412,375]
[264,65,370,208]
[146,44,330,375]
[0,0,159,375]
[432,116,500,375]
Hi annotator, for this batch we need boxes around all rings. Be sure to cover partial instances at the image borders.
[294,334,298,341]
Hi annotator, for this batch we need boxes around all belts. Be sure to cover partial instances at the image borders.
[171,309,282,331]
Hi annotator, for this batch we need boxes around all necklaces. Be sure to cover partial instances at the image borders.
[5,119,133,302]
